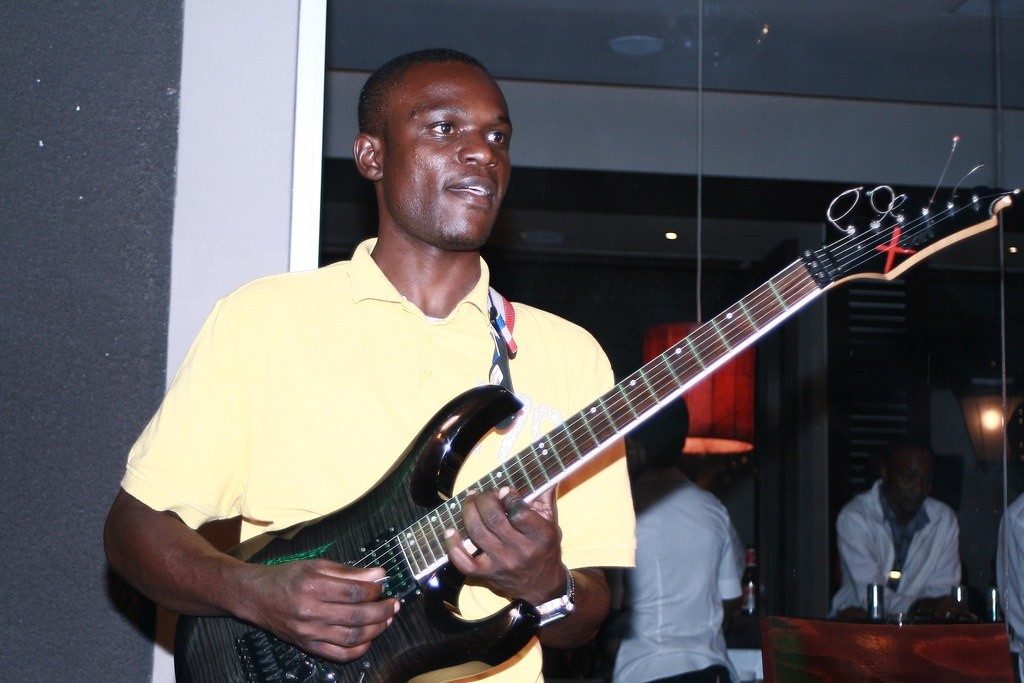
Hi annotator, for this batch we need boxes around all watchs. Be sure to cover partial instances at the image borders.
[533,561,575,627]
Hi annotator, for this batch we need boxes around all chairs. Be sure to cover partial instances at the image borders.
[758,615,1018,683]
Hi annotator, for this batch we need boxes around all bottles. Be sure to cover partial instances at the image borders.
[739,548,762,618]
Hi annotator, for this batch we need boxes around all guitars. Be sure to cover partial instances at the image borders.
[169,180,1022,682]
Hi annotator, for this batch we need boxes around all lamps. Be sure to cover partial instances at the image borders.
[640,0,753,454]
[936,358,1024,472]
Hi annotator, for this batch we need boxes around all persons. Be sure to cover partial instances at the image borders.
[995,401,1024,683]
[612,397,746,683]
[826,439,962,625]
[103,47,637,683]
